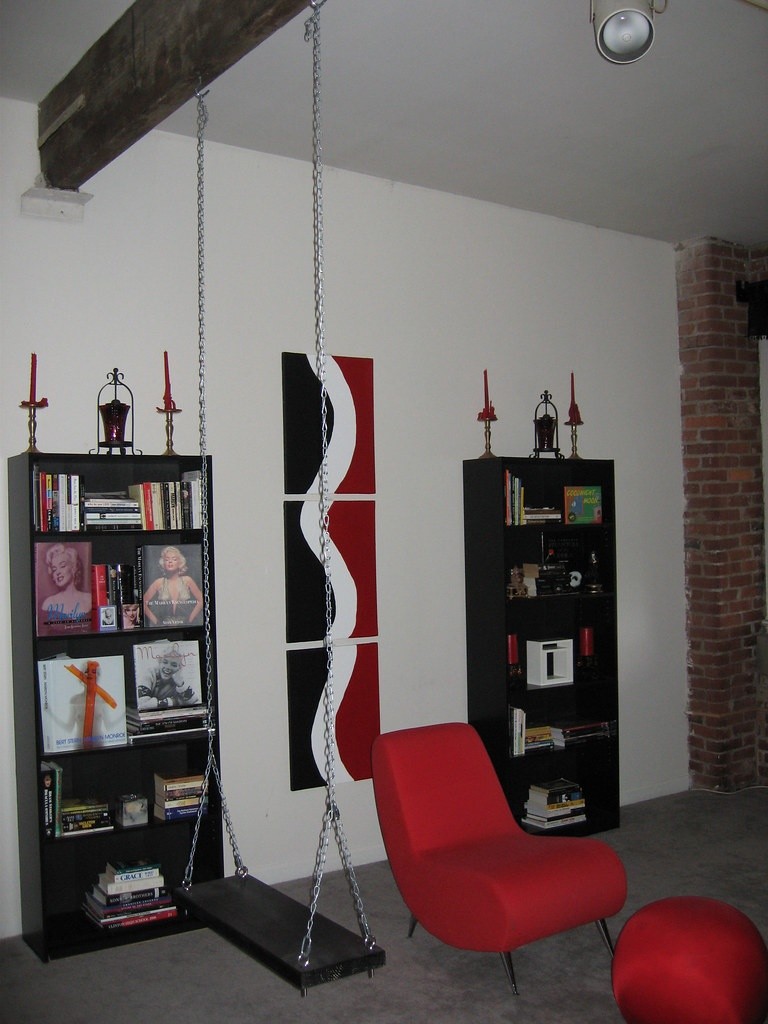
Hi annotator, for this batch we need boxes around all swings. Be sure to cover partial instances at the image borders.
[171,0,390,998]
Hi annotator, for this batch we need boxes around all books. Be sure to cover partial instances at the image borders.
[79,859,178,930]
[509,704,554,757]
[153,771,210,820]
[32,541,92,636]
[86,491,143,530]
[127,638,209,745]
[39,761,113,838]
[522,777,586,829]
[138,543,204,627]
[32,462,85,531]
[549,721,609,746]
[564,486,603,524]
[504,468,561,526]
[129,470,203,530]
[92,563,139,632]
[37,655,127,754]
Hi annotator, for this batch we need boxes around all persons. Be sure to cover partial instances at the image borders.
[145,545,203,625]
[123,604,138,628]
[40,545,92,628]
[137,651,199,709]
[101,607,114,625]
[45,661,125,747]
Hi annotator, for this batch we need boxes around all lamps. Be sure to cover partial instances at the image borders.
[589,0,668,66]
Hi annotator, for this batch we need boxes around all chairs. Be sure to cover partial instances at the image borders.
[371,722,628,996]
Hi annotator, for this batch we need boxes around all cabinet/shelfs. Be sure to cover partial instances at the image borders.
[462,457,621,839]
[7,451,225,965]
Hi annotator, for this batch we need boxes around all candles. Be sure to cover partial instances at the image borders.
[571,369,576,421]
[30,351,37,403]
[483,368,490,412]
[163,349,173,410]
